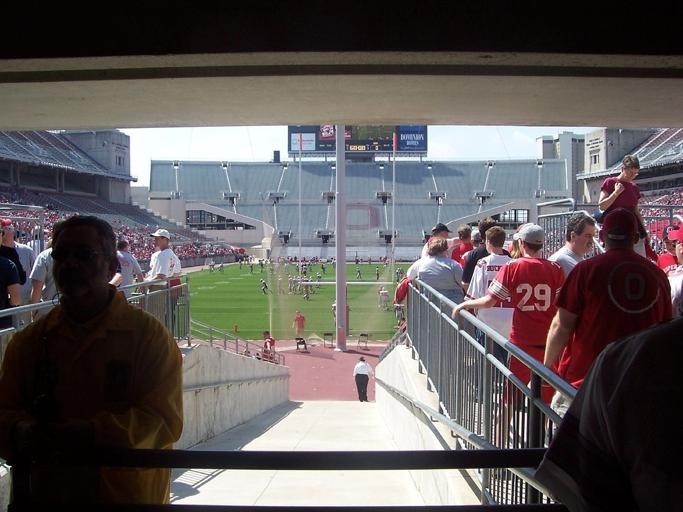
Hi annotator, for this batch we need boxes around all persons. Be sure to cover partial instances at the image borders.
[352,356,374,403]
[378,286,389,311]
[367,256,371,264]
[359,257,363,263]
[382,261,388,272]
[355,257,358,264]
[376,285,383,309]
[354,266,361,280]
[246,252,336,301]
[329,298,335,329]
[0,187,245,323]
[261,331,275,362]
[382,256,385,263]
[290,310,305,341]
[375,265,379,281]
[0,213,186,511]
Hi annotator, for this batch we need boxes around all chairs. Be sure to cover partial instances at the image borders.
[355,333,369,352]
[294,337,307,351]
[0,185,243,265]
[322,331,333,349]
[526,127,682,265]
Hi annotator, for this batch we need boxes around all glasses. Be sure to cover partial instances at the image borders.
[53,241,111,262]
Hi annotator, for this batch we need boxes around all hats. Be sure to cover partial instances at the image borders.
[149,228,170,241]
[667,224,683,243]
[512,222,546,246]
[431,223,453,234]
[601,206,638,239]
